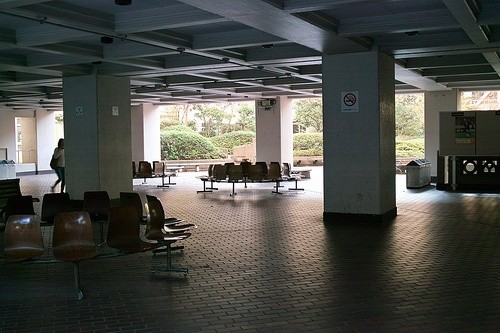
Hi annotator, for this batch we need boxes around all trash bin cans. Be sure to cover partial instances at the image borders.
[406,159,432,187]
[0,160,8,180]
[6,160,16,179]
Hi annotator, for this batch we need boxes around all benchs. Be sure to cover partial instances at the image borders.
[165,162,220,172]
[292,167,311,178]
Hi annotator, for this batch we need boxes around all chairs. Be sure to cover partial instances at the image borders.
[0,191,197,300]
[197,162,305,196]
[132,161,176,188]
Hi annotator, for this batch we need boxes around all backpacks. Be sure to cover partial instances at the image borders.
[50,154,57,169]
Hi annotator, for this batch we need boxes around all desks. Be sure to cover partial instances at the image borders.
[0,163,16,180]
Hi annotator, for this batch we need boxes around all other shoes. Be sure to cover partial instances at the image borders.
[61,191,64,193]
[51,187,54,192]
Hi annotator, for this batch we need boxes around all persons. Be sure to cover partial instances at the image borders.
[50,138,66,193]
